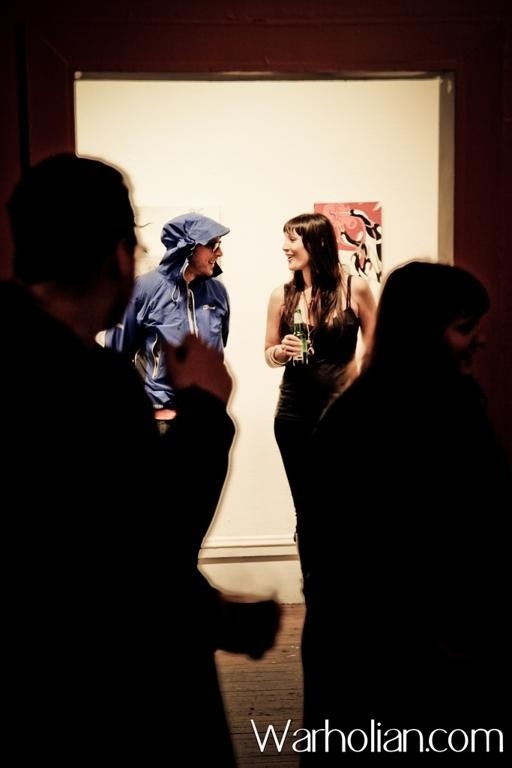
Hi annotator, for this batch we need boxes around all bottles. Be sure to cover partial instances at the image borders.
[290,309,309,368]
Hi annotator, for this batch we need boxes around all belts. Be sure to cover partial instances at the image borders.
[154,409,177,420]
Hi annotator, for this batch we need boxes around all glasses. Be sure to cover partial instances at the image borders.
[208,241,222,253]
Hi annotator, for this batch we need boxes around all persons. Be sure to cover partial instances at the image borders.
[264,213,378,480]
[0,155,279,768]
[299,260,512,768]
[105,211,231,434]
